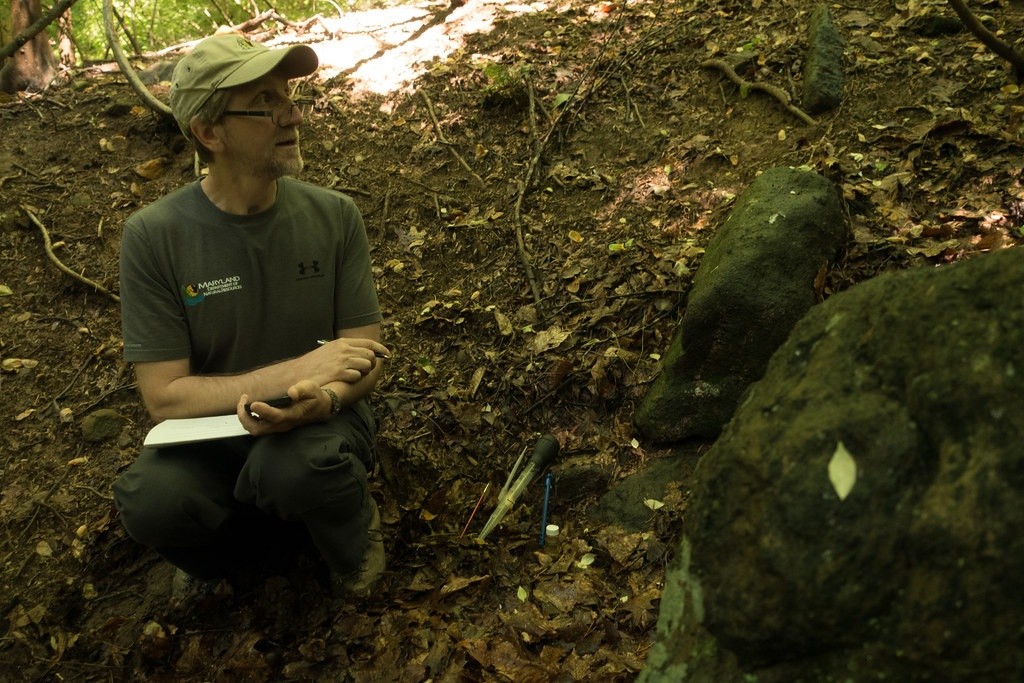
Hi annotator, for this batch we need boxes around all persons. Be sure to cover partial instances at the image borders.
[111,33,390,619]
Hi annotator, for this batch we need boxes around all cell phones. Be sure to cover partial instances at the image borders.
[243,393,292,412]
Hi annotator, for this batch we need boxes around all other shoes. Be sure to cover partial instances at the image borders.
[336,498,385,597]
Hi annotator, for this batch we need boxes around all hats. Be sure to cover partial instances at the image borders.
[171,33,319,138]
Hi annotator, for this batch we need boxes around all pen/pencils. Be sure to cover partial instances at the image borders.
[317,339,391,360]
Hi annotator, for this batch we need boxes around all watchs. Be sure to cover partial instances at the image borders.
[323,388,339,421]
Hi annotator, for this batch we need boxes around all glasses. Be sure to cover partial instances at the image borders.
[223,99,316,126]
